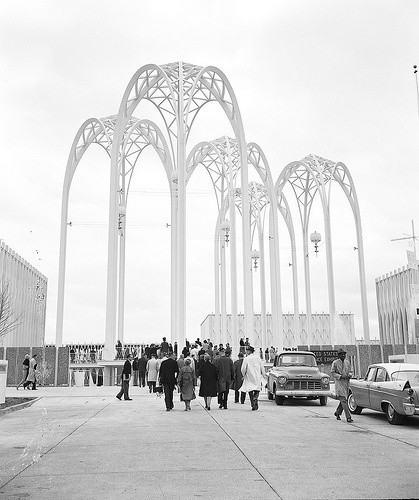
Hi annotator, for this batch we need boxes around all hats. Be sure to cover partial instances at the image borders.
[338,348,347,354]
[238,353,244,357]
[246,346,255,351]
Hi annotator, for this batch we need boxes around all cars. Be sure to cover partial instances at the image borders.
[265,351,331,407]
[348,363,419,425]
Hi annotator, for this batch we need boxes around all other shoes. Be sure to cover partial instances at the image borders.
[124,398,132,400]
[116,395,122,400]
[347,418,353,422]
[163,400,258,411]
[16,385,38,390]
[334,412,340,420]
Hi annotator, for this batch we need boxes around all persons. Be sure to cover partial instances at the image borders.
[240,338,250,355]
[138,353,148,387]
[124,345,161,359]
[238,346,265,411]
[331,349,353,422]
[116,354,132,400]
[216,352,234,409]
[259,346,278,363]
[17,354,30,390]
[70,345,104,363]
[233,353,246,404]
[23,354,38,390]
[114,340,123,360]
[159,353,180,411]
[197,355,218,410]
[178,359,197,411]
[133,357,140,386]
[146,354,159,393]
[161,339,231,386]
[161,338,170,358]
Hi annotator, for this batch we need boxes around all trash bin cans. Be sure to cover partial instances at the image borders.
[0,360,9,405]
[74,372,85,387]
